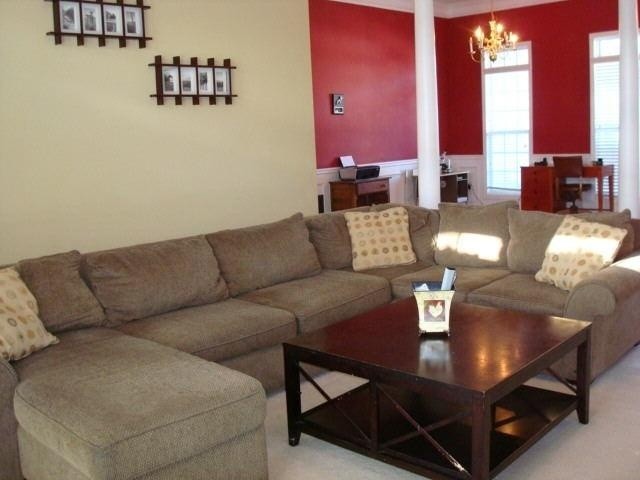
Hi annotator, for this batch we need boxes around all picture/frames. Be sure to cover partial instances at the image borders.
[148,55,237,105]
[44,0,152,48]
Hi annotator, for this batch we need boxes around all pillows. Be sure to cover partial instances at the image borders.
[0,266,60,361]
[343,206,417,272]
[535,215,628,292]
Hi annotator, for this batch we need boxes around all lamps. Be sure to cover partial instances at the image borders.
[469,1,517,64]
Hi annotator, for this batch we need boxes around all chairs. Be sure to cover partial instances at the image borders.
[553,156,592,215]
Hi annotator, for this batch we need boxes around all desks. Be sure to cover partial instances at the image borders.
[521,164,615,213]
[417,172,469,204]
[328,176,391,212]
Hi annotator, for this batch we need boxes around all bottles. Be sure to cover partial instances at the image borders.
[543,158,548,166]
[440,156,450,169]
[598,158,603,166]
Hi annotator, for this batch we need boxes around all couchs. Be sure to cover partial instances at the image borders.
[0,202,640,480]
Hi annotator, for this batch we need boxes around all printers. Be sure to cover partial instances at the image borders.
[339,156,380,181]
